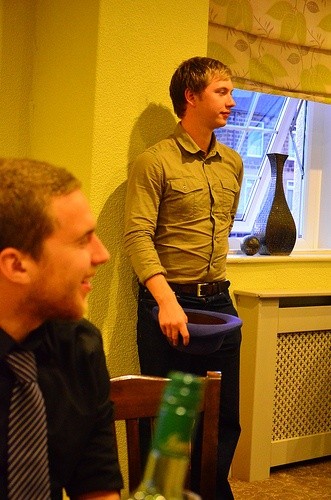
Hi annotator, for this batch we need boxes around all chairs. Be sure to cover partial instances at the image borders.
[110,370,219,500]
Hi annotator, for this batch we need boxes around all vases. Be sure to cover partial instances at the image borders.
[251,152,298,256]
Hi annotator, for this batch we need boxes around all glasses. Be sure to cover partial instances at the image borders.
[167,281,230,297]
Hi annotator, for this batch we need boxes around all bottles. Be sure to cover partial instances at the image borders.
[126,371,203,500]
[251,153,296,256]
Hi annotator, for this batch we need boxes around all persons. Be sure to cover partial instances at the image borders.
[123,56,242,500]
[0,158,124,500]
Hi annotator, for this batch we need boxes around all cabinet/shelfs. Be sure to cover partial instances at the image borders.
[232,288,331,485]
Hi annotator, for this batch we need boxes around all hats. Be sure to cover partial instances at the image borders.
[153,305,241,355]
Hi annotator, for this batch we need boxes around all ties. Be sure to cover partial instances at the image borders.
[7,351,51,500]
